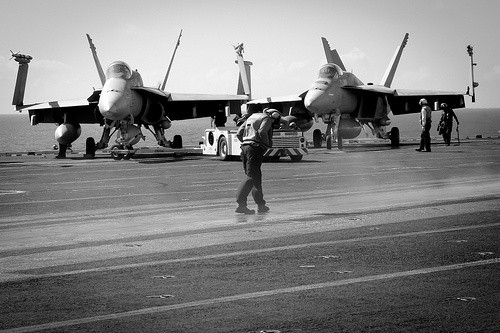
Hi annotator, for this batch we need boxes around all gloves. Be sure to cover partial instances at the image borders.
[422,127,425,132]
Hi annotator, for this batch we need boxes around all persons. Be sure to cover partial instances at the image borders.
[415,98,432,152]
[235,108,281,215]
[437,103,459,146]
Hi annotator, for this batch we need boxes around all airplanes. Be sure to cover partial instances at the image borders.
[233,33,478,150]
[8,28,250,160]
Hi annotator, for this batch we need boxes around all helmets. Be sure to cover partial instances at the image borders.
[419,98,427,105]
[441,103,447,108]
[264,108,281,130]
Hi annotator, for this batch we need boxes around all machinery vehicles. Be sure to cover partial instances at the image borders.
[201,113,309,162]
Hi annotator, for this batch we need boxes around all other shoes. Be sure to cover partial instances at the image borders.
[415,149,422,152]
[236,206,254,214]
[258,206,269,213]
[422,149,431,152]
